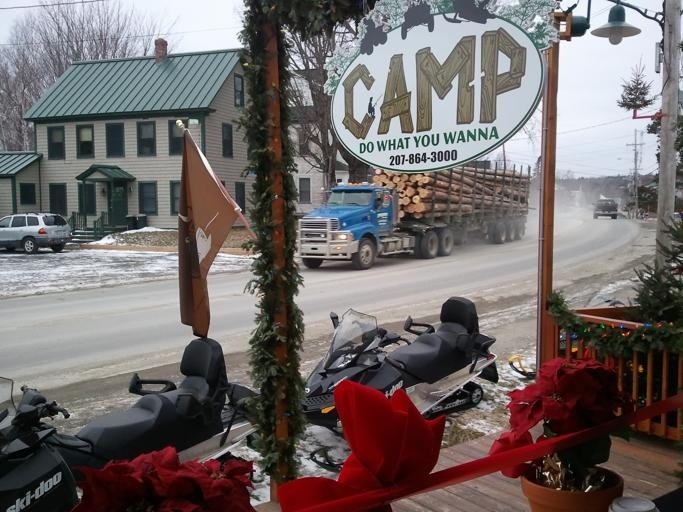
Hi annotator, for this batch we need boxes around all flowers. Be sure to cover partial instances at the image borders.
[71,447,259,512]
[494,356,640,492]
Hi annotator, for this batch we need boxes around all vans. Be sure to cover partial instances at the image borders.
[0,211,72,254]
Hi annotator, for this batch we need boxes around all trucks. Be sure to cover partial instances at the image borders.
[296,162,532,272]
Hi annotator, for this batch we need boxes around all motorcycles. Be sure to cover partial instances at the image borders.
[297,295,499,441]
[0,337,262,511]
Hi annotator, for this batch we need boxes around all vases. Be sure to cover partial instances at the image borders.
[521,464,624,511]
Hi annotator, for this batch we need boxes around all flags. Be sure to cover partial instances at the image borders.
[171,131,240,339]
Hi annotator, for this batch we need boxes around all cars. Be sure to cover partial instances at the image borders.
[591,198,618,219]
